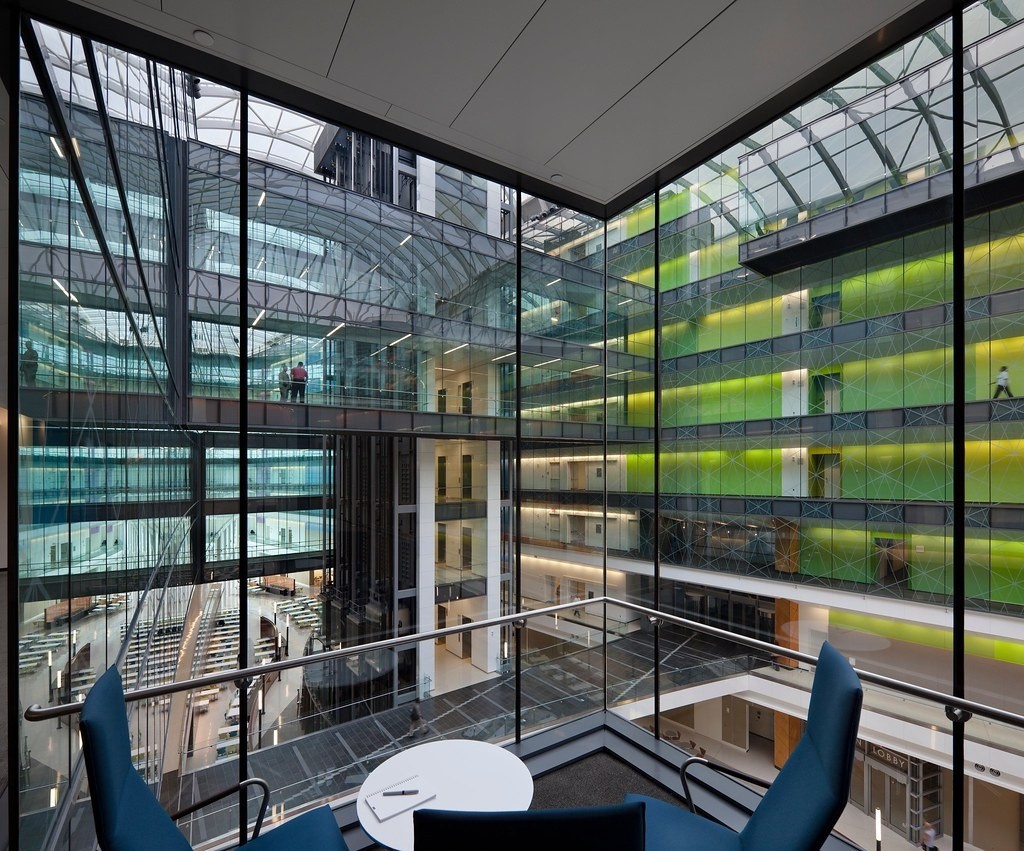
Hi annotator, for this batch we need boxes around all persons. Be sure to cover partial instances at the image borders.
[279,365,290,401]
[922,823,935,851]
[464,306,473,323]
[409,697,429,738]
[994,366,1014,399]
[20,341,38,387]
[291,362,308,403]
[572,594,581,617]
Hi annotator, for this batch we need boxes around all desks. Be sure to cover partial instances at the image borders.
[354,738,535,851]
[194,578,322,759]
[662,729,676,738]
[676,741,693,750]
[18,591,175,774]
[686,748,700,757]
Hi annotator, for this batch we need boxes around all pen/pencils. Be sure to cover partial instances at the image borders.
[383,789,420,797]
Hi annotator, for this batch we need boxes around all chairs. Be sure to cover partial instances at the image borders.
[79,663,349,850]
[412,802,646,851]
[626,639,865,851]
[649,725,706,759]
